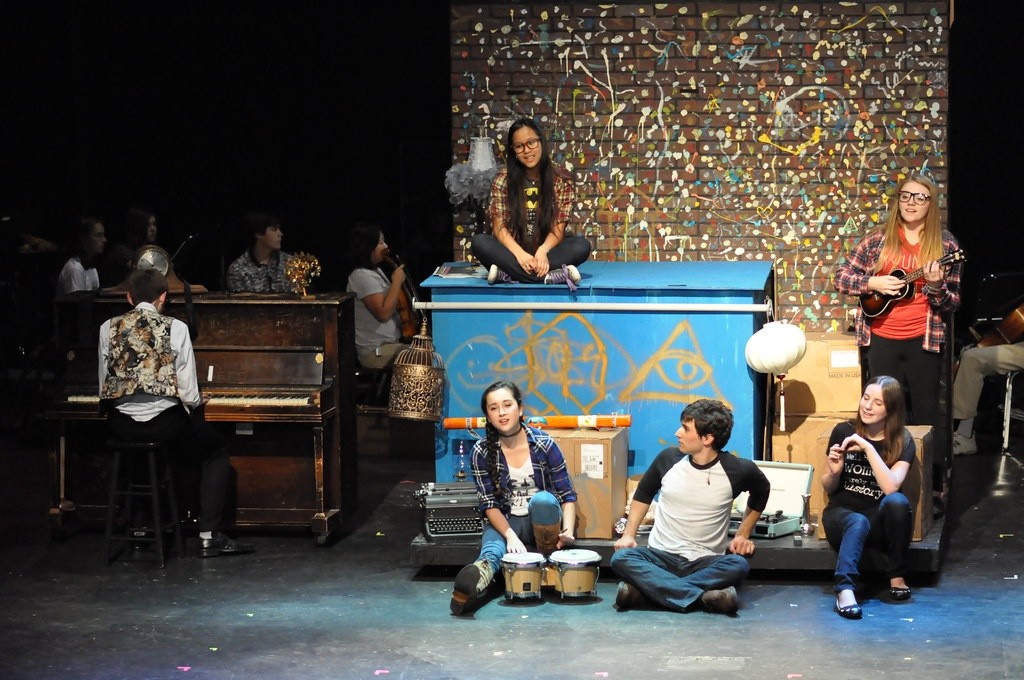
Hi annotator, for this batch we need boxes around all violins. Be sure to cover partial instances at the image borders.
[380,247,425,338]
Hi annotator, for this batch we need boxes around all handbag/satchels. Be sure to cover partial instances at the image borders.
[345,368,394,403]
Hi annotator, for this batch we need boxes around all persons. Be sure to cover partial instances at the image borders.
[450,381,577,617]
[951,342,1024,455]
[834,174,964,516]
[610,398,770,615]
[98,271,255,557]
[470,118,590,285]
[55,215,107,297]
[343,221,418,369]
[103,209,159,288]
[227,210,296,291]
[819,376,916,617]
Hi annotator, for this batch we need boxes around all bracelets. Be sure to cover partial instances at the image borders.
[500,427,522,437]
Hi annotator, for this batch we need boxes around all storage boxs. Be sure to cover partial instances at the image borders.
[541,428,631,540]
[771,333,934,542]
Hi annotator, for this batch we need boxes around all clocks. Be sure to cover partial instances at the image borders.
[101,245,208,297]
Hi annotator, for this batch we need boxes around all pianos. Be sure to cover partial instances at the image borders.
[40,289,358,545]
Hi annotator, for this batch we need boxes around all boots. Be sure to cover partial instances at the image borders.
[530,518,560,557]
[450,556,494,616]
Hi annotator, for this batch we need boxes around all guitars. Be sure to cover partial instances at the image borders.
[858,247,965,319]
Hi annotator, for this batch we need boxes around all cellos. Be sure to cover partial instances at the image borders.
[976,304,1024,348]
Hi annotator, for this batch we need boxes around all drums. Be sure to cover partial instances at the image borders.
[548,550,602,597]
[498,551,547,600]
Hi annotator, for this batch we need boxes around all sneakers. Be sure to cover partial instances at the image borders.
[544,264,580,292]
[488,264,520,284]
[953,431,978,455]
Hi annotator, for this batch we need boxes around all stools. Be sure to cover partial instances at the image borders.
[1003,370,1024,450]
[101,437,184,567]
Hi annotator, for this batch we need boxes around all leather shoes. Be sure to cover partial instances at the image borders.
[890,587,910,598]
[616,581,648,607]
[702,586,740,614]
[131,534,155,552]
[196,533,256,557]
[836,596,863,618]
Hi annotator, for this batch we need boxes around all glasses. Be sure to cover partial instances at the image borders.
[510,137,542,154]
[898,189,932,205]
